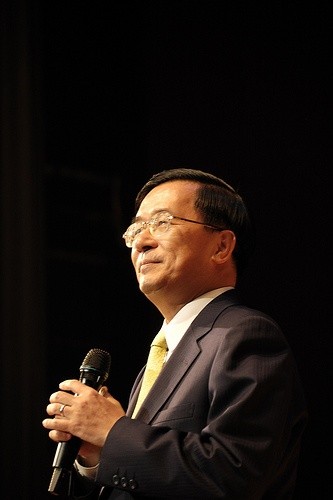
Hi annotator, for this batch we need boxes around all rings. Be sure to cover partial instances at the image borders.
[60,404,65,416]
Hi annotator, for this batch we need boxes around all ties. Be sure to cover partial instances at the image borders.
[131,331,167,420]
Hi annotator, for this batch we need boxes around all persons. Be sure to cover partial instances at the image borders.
[42,167,316,500]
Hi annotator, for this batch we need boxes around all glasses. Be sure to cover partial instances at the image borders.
[123,212,227,249]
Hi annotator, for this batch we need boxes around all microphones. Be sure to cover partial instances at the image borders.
[47,348,111,497]
